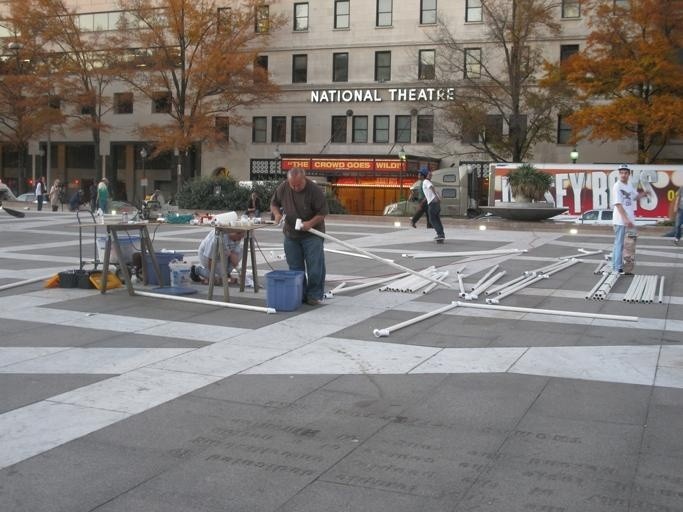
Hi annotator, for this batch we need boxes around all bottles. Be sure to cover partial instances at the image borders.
[96,207,104,226]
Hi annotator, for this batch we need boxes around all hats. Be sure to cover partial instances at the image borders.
[618,163,632,172]
[416,166,429,177]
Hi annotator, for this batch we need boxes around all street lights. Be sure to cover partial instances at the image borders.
[567,148,579,164]
[140,147,148,202]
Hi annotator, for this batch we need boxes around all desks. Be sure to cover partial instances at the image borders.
[72,220,269,303]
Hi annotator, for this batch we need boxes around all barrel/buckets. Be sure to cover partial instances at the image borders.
[143,250,185,286]
[168,258,191,289]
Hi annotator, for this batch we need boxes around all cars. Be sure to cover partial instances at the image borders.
[106,200,138,215]
[568,207,612,225]
[0,192,50,209]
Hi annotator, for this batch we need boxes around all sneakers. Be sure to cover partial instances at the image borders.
[190,265,202,283]
[673,237,681,243]
[433,236,446,239]
[426,225,435,229]
[409,221,417,229]
[307,299,322,306]
[618,269,625,275]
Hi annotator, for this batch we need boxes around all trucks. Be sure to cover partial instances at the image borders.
[381,159,683,220]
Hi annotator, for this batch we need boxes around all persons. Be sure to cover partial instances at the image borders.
[87,178,97,213]
[410,170,433,230]
[34,175,46,211]
[610,162,649,275]
[48,179,62,211]
[268,167,328,305]
[188,227,245,285]
[66,188,83,211]
[672,185,682,244]
[242,192,260,218]
[416,165,445,240]
[97,176,111,213]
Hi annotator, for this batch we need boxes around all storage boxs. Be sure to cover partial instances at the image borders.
[143,252,184,283]
[95,235,141,264]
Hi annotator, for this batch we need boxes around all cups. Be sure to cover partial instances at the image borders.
[121,211,128,223]
